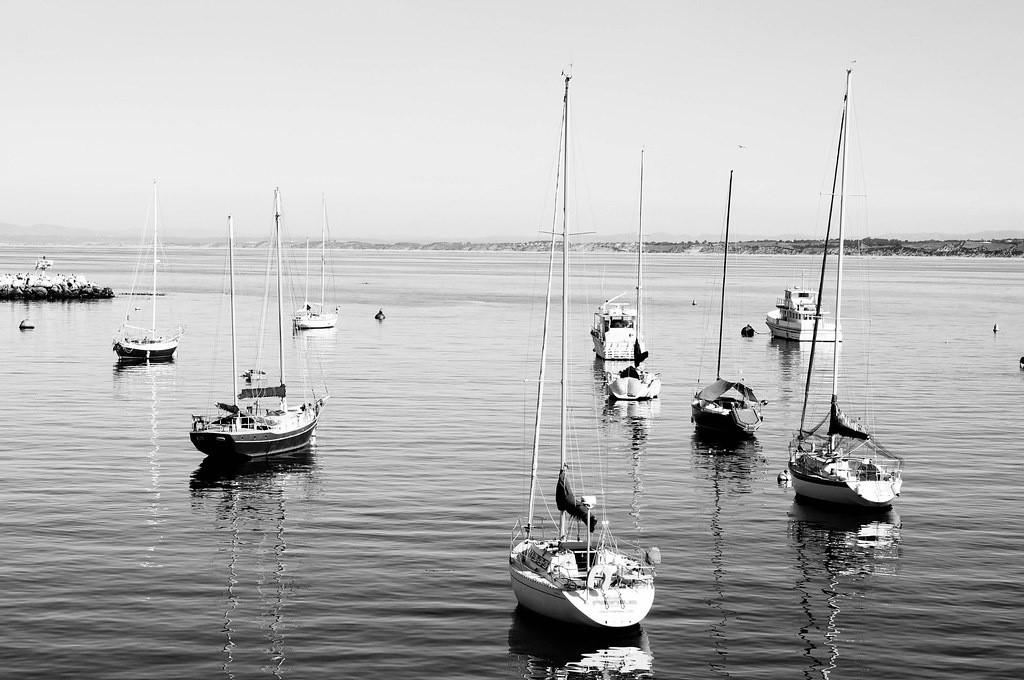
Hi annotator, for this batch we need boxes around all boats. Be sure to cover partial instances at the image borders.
[767,285,842,342]
[588,294,645,362]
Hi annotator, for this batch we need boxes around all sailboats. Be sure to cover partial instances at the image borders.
[787,63,906,507]
[602,147,660,401]
[187,184,332,462]
[690,167,769,434]
[508,65,662,628]
[291,194,340,330]
[112,181,186,359]
